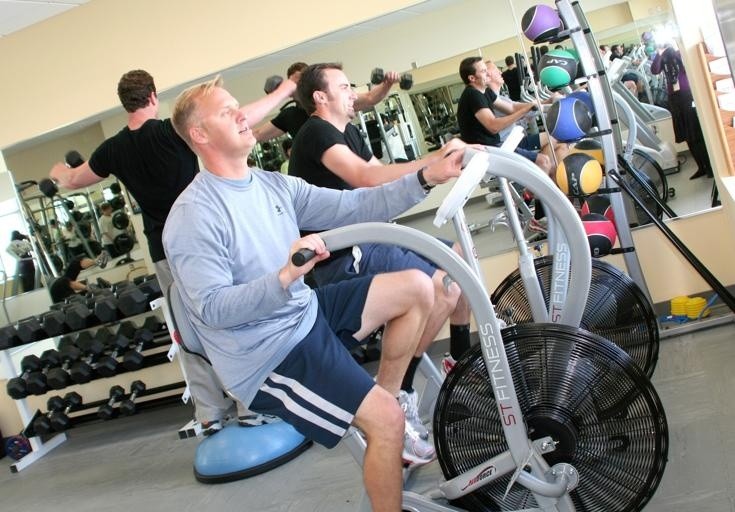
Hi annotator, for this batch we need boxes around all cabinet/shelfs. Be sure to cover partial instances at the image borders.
[0,296,200,474]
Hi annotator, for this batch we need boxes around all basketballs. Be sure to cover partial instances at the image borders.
[581,197,616,225]
[645,44,655,55]
[556,153,604,194]
[520,5,560,41]
[547,97,591,141]
[580,212,616,257]
[537,49,578,88]
[570,139,605,172]
[642,32,656,42]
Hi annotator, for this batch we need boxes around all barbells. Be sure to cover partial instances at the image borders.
[43,182,133,274]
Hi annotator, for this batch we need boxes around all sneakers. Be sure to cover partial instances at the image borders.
[440,352,457,377]
[237,412,282,426]
[528,214,548,234]
[371,372,437,465]
[199,413,234,437]
[503,204,536,221]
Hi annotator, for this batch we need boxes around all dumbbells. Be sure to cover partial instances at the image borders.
[263,78,283,93]
[371,67,414,89]
[39,151,87,196]
[0,272,164,434]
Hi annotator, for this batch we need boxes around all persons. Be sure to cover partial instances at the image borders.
[599,26,714,181]
[9,230,36,293]
[289,63,488,439]
[162,72,472,512]
[251,63,413,141]
[39,70,297,436]
[50,251,109,304]
[50,204,136,261]
[456,56,555,233]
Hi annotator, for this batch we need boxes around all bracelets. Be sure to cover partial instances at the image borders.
[418,166,436,191]
